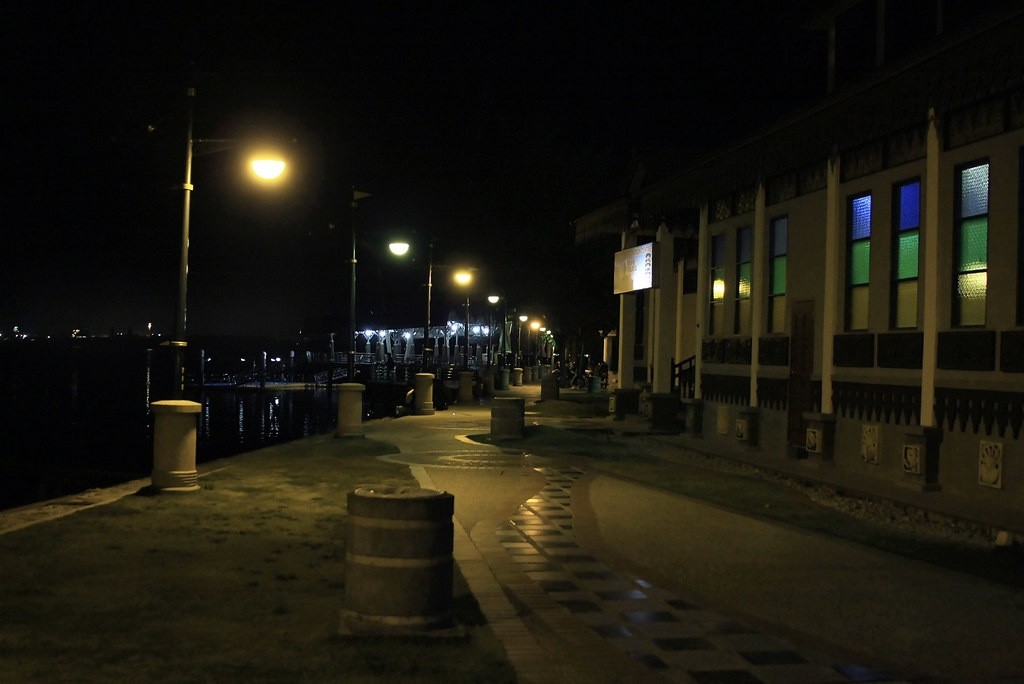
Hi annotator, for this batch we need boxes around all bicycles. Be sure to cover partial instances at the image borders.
[551,362,609,389]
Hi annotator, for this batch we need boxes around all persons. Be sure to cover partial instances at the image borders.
[599,360,608,381]
[552,361,569,377]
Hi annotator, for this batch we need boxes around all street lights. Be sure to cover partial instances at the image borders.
[175,77,285,395]
[485,288,503,367]
[517,312,528,367]
[424,241,479,371]
[530,319,555,366]
[345,207,413,384]
